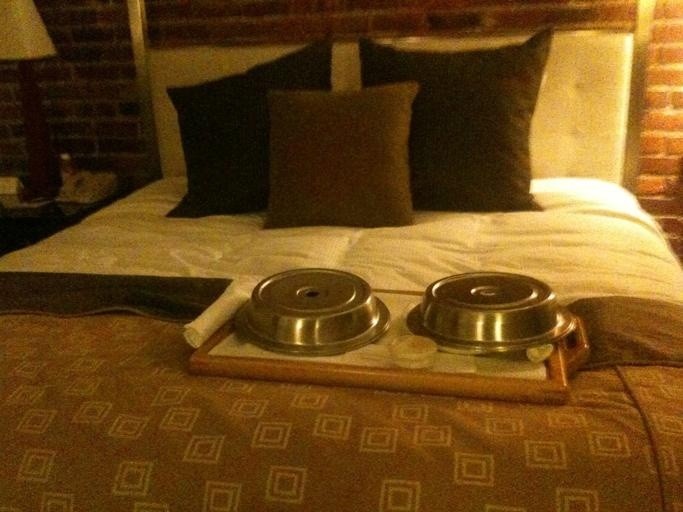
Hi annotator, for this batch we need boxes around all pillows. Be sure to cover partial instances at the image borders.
[354,22,550,215]
[163,38,336,218]
[258,81,420,233]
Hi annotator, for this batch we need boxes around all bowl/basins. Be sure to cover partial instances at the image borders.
[404,269,578,356]
[390,335,435,368]
[231,267,389,356]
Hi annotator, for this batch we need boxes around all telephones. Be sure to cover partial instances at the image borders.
[59,169,119,205]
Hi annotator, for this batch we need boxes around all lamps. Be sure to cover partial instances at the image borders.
[0,0,66,197]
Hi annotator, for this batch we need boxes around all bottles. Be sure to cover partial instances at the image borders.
[59,153,72,184]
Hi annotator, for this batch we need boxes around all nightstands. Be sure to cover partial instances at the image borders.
[0,176,132,256]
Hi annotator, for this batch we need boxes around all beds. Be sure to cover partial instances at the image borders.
[0,1,683,511]
